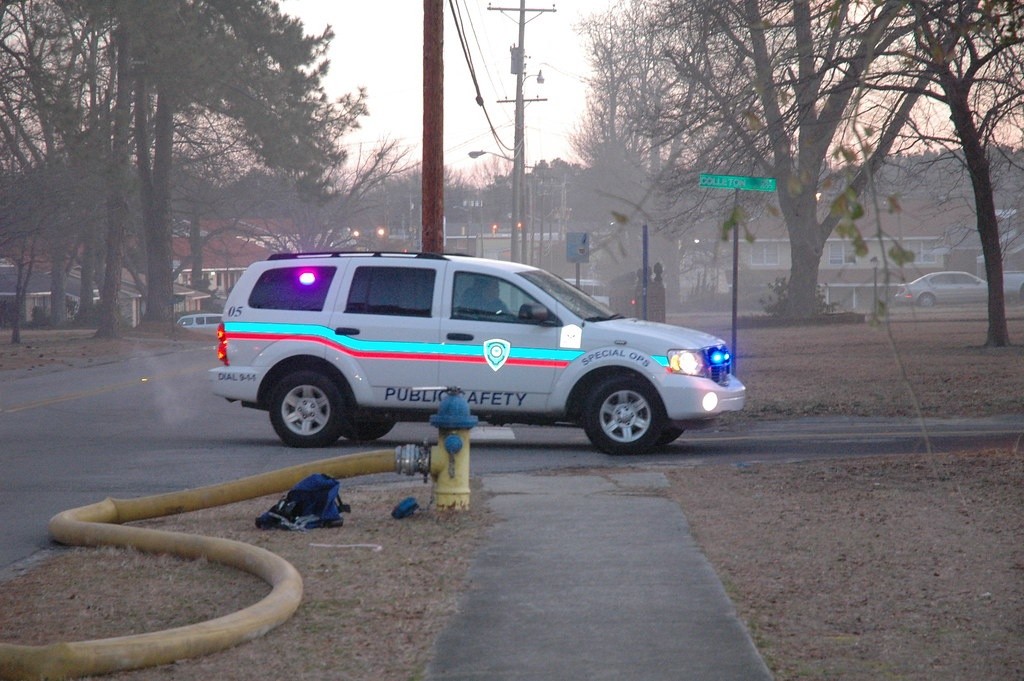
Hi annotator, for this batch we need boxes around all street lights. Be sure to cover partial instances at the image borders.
[468,149,525,264]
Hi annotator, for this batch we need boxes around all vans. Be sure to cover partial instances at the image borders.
[177,314,222,337]
[560,278,608,309]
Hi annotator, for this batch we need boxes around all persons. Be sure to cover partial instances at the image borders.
[457,274,513,320]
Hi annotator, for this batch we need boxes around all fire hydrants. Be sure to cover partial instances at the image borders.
[415,386,481,513]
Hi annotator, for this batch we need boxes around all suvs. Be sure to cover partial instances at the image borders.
[207,248,748,457]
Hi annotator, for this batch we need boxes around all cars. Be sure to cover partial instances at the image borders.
[895,270,989,308]
